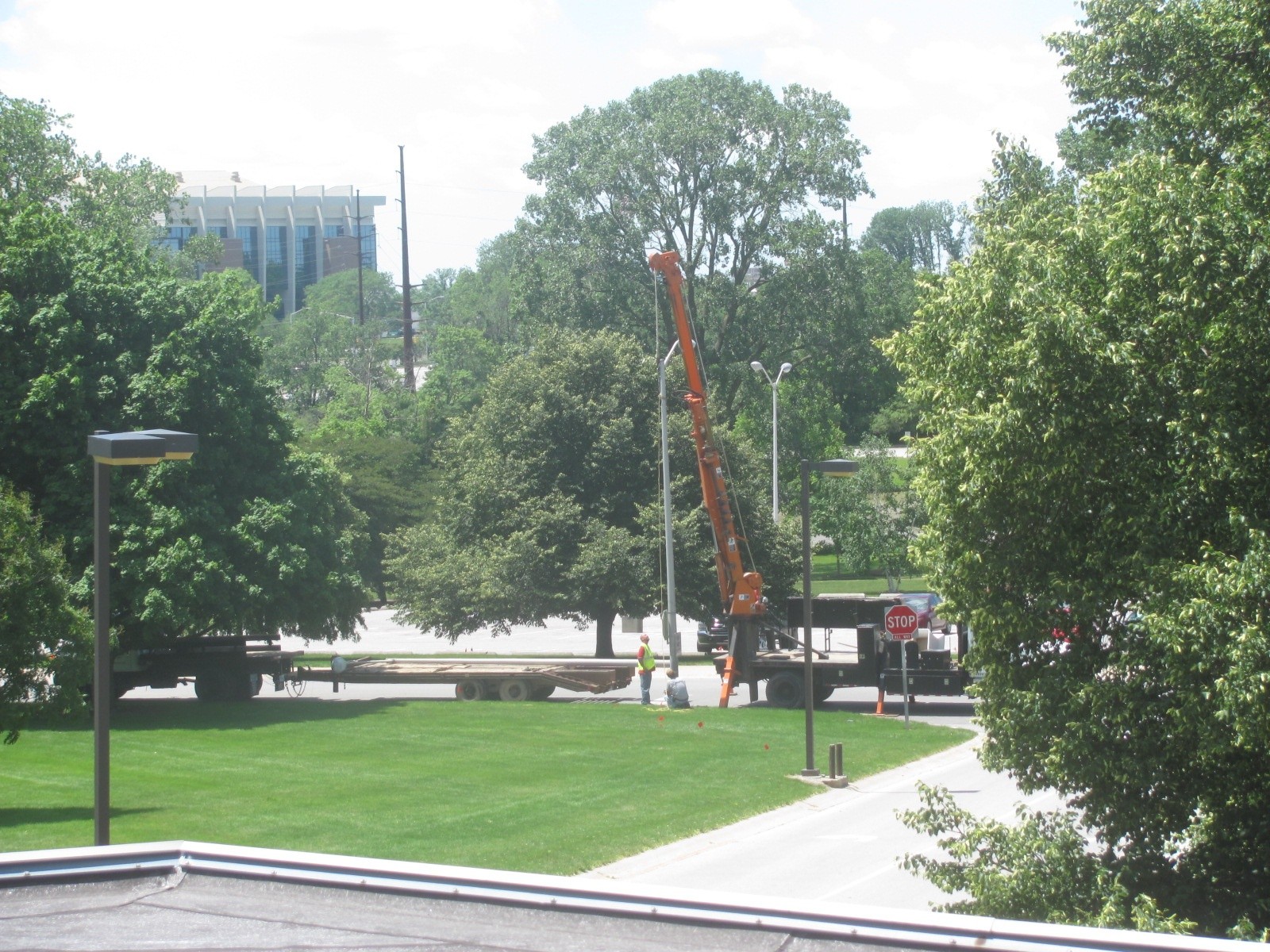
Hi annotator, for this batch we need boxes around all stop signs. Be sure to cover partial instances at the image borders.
[885,605,919,637]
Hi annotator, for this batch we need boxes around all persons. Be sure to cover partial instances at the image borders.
[636,633,655,704]
[663,669,690,707]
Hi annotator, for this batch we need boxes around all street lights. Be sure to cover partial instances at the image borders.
[656,337,697,676]
[82,429,206,848]
[749,359,793,522]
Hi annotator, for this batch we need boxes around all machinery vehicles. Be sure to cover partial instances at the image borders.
[645,249,800,654]
